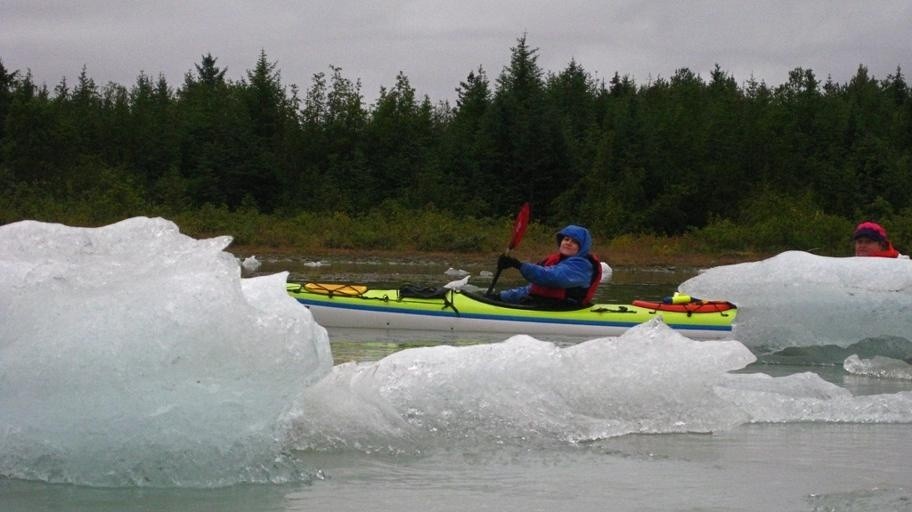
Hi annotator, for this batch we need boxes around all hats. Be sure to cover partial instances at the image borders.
[851,221,887,242]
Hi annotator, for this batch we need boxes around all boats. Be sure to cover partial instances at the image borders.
[284,282,739,340]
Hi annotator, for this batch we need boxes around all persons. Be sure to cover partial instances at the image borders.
[485,225,602,306]
[853,222,898,257]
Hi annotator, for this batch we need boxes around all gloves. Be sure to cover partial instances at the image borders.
[497,253,519,270]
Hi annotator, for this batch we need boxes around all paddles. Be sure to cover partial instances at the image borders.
[485,203,529,298]
